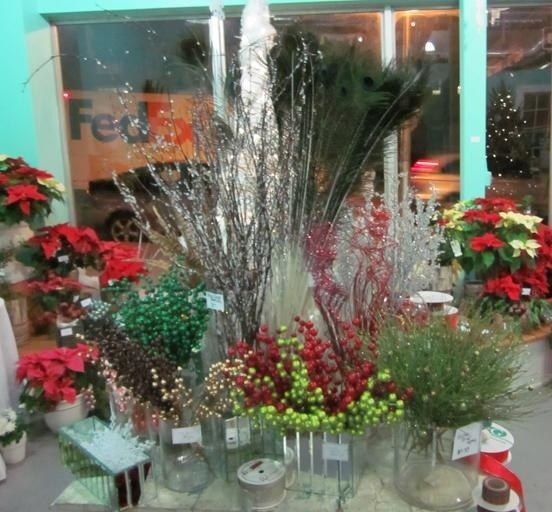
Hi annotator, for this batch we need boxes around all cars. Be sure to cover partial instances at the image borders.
[88,162,221,244]
[409,154,547,211]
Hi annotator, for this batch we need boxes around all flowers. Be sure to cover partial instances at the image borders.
[1,156,147,445]
[428,194,552,333]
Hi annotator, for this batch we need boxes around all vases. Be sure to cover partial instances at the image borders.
[1,431,28,464]
[151,412,370,497]
[391,416,482,510]
